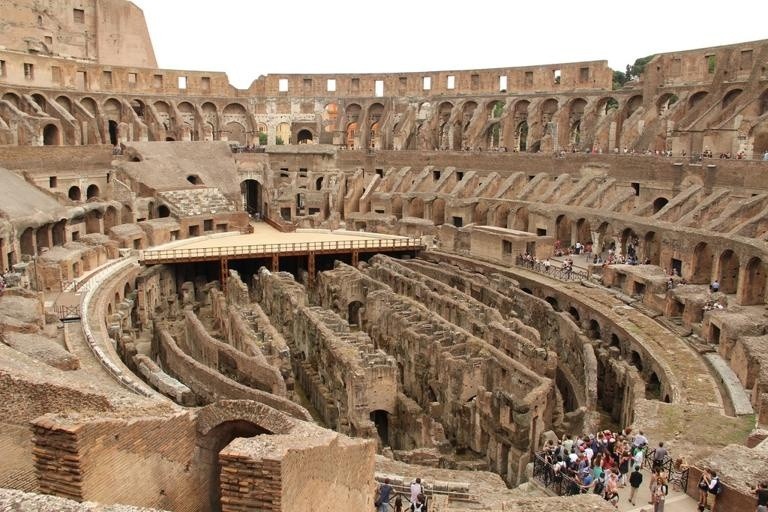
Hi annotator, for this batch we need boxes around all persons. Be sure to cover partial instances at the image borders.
[697,467,722,512]
[233,142,258,152]
[749,482,768,512]
[760,149,768,161]
[440,145,520,153]
[519,242,724,314]
[542,425,690,512]
[375,478,427,512]
[0,268,14,289]
[248,211,263,221]
[555,144,747,161]
